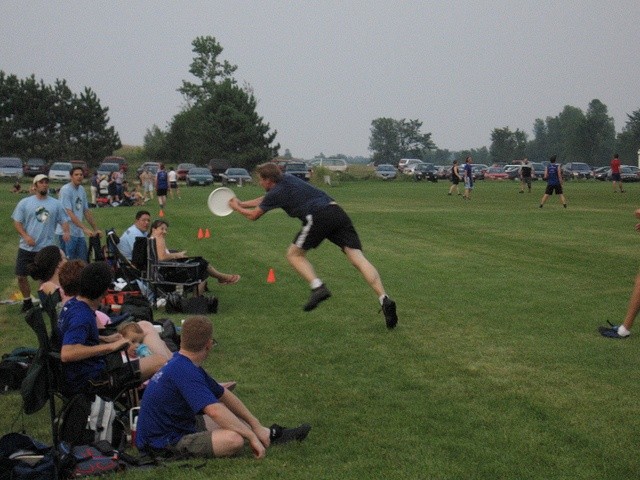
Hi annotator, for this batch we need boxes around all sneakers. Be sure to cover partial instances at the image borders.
[304,282,333,311]
[600,320,631,338]
[378,296,399,330]
[271,423,313,446]
[20,298,34,313]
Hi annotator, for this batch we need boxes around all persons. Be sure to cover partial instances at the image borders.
[117,321,147,362]
[58,261,112,329]
[154,163,171,209]
[32,245,69,295]
[448,159,462,196]
[56,168,103,260]
[117,211,150,261]
[57,261,236,400]
[90,173,98,203]
[148,220,240,285]
[611,152,625,193]
[11,174,69,312]
[229,163,398,330]
[167,166,182,199]
[463,156,474,200]
[136,316,311,459]
[599,207,640,337]
[108,165,153,206]
[540,156,566,208]
[518,157,535,193]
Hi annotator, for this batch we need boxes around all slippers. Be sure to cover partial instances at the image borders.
[221,381,237,391]
[221,274,241,285]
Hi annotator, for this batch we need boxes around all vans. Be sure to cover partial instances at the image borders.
[207,158,231,181]
[398,159,424,173]
[310,158,348,172]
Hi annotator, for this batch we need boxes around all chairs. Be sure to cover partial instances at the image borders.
[106,231,180,293]
[86,234,104,262]
[38,292,160,358]
[140,235,202,312]
[21,299,168,467]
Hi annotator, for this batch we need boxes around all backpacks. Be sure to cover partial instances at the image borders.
[70,440,141,479]
[120,293,154,322]
[1,432,80,479]
[1,345,39,395]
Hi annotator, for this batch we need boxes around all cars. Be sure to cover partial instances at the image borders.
[626,166,640,176]
[414,163,439,183]
[102,156,128,172]
[284,161,311,181]
[96,163,120,175]
[70,160,89,177]
[438,167,451,179]
[503,165,521,179]
[48,162,73,183]
[186,167,214,186]
[0,157,24,182]
[271,160,287,170]
[403,163,417,178]
[596,166,610,180]
[608,167,640,182]
[593,167,610,176]
[137,162,160,175]
[376,164,396,180]
[447,166,478,180]
[563,162,593,179]
[465,164,489,179]
[221,168,252,186]
[484,167,509,179]
[176,163,196,180]
[530,162,548,180]
[24,158,49,177]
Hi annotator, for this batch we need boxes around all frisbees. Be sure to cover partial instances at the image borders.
[207,187,236,217]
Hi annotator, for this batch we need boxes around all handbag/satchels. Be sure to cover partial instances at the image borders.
[182,295,210,314]
[20,338,54,415]
[102,291,141,313]
[165,290,185,313]
[207,295,219,313]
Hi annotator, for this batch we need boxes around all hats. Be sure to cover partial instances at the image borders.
[33,174,49,185]
